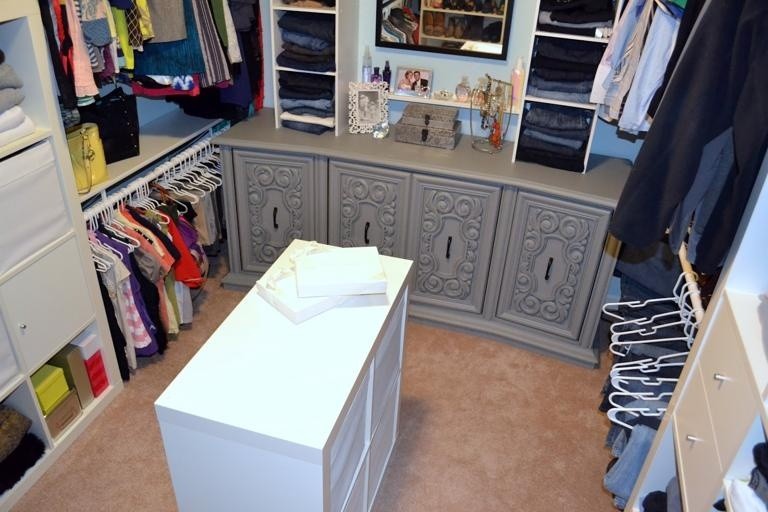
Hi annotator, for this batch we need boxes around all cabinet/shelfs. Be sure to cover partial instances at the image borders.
[418,0,508,52]
[329,133,411,295]
[621,150,768,512]
[0,0,124,512]
[482,141,634,365]
[270,0,624,175]
[214,106,329,292]
[415,134,504,338]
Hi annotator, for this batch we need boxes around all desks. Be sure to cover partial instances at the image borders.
[154,238,414,512]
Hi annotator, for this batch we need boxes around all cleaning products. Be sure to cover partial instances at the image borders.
[361,47,373,84]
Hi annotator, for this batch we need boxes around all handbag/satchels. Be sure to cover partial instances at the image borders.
[67,123,109,190]
[80,88,140,164]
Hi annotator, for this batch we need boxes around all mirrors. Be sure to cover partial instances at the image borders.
[375,0,514,61]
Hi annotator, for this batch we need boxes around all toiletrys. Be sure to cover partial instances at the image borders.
[455,77,471,103]
[370,67,383,88]
[382,61,392,92]
[471,78,486,105]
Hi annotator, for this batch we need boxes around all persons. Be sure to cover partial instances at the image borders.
[358,92,379,123]
[398,70,413,90]
[411,71,428,91]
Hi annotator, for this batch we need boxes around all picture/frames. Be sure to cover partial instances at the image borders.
[348,81,388,134]
[394,65,433,97]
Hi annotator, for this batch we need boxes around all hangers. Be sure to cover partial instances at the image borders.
[83,121,230,272]
[602,271,705,430]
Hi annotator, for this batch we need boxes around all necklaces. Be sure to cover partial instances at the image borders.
[478,79,508,148]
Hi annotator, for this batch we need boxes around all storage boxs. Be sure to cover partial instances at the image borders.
[30,330,109,439]
